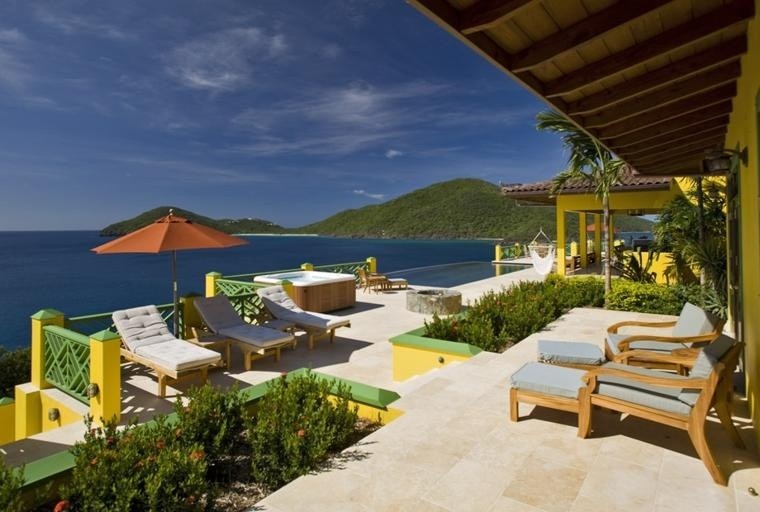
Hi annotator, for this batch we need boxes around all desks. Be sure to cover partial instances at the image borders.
[187,333,239,373]
[262,317,296,351]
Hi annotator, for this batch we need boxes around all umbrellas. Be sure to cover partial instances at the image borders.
[91,208,250,339]
[586,223,616,239]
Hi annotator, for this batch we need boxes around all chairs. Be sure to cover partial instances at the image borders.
[357,266,389,294]
[110,304,222,399]
[255,284,350,351]
[576,335,747,487]
[604,302,729,374]
[186,293,296,371]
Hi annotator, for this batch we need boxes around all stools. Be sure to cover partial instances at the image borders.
[535,339,604,371]
[508,361,592,427]
[388,278,408,291]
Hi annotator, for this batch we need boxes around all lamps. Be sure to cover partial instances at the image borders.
[704,146,746,176]
